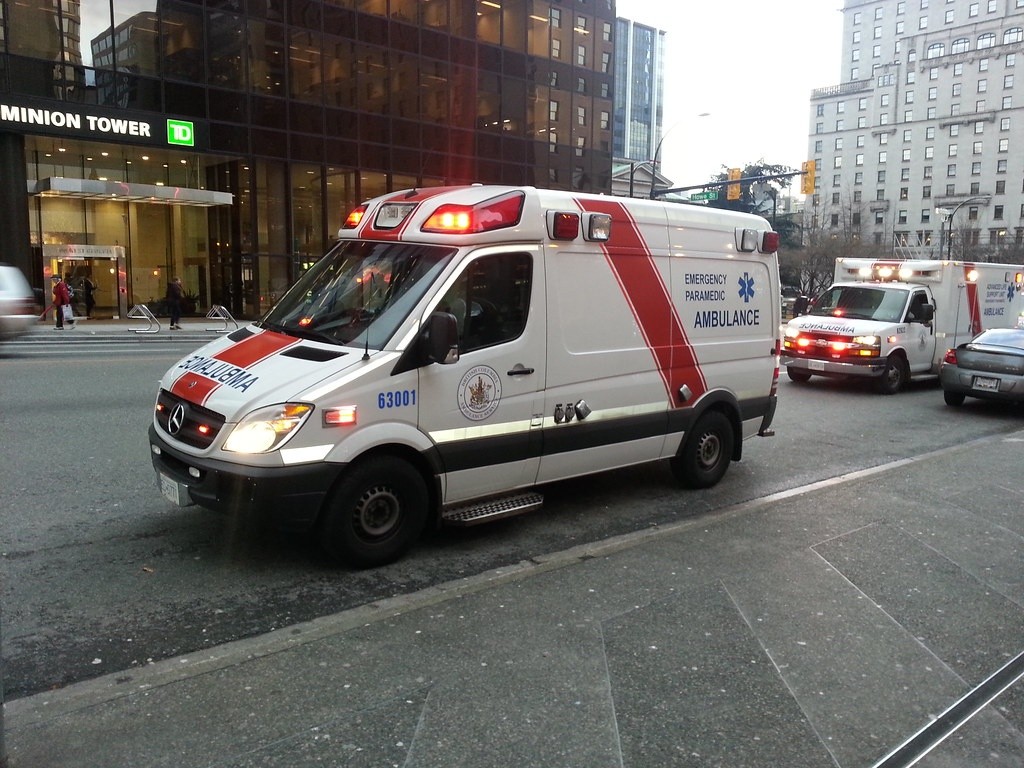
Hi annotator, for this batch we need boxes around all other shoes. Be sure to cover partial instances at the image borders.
[170,327,177,330]
[54,326,64,330]
[66,324,76,330]
[176,325,182,329]
[87,317,95,320]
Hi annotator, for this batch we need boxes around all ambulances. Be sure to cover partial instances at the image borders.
[147,182,778,570]
[777,255,1024,396]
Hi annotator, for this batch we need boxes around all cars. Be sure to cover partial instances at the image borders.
[779,285,810,315]
[938,327,1024,408]
[0,263,42,342]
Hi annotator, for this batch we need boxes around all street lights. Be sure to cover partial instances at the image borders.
[629,161,661,198]
[651,111,710,200]
[947,194,993,260]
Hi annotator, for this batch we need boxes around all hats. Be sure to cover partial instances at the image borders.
[50,274,62,279]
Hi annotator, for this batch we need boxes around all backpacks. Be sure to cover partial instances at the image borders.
[66,285,75,299]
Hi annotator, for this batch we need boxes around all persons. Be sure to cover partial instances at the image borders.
[83,271,99,319]
[50,274,75,330]
[166,276,185,330]
[793,291,809,318]
[63,272,78,317]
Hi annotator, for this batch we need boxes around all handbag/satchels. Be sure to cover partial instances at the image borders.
[62,304,73,320]
